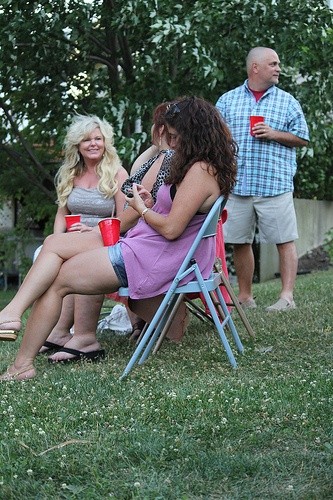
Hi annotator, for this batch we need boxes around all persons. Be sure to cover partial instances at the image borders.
[216,46,311,312]
[0,95,240,381]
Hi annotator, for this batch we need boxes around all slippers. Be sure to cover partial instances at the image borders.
[36,341,65,357]
[48,346,106,366]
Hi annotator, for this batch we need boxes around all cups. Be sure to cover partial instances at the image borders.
[65,215,81,233]
[249,115,265,136]
[98,218,121,247]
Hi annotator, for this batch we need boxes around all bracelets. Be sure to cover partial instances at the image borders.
[142,208,149,223]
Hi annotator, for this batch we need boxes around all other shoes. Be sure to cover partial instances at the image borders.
[239,296,258,311]
[265,295,296,313]
[127,321,147,344]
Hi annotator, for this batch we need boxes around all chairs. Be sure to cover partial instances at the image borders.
[116,192,257,378]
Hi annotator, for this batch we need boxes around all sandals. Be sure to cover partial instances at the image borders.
[0,320,22,341]
[0,365,38,382]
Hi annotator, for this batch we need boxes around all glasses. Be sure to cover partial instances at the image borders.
[169,103,180,114]
